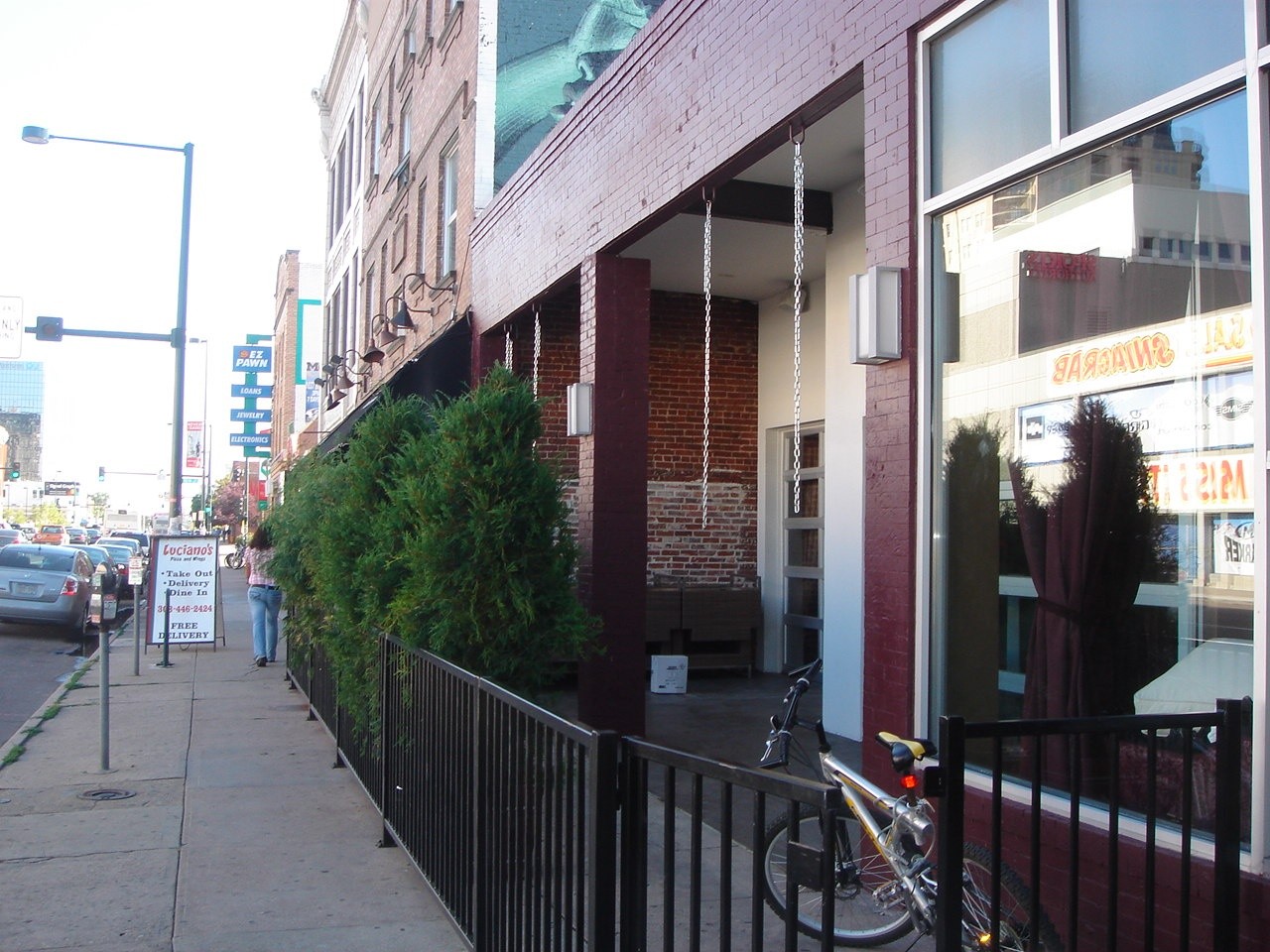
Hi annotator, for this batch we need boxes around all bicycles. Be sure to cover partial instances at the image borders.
[759,658,1065,952]
[223,540,250,570]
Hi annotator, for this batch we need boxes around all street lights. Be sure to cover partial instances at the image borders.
[20,124,194,531]
[190,338,209,522]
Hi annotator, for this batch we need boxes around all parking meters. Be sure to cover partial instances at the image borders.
[99,573,122,773]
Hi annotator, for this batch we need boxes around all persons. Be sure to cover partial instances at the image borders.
[245,524,284,667]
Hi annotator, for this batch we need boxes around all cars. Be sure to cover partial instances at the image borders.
[0,520,200,632]
[0,543,110,636]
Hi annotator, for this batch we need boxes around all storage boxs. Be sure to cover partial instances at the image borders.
[651,654,690,693]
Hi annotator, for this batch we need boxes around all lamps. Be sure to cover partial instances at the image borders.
[361,313,410,363]
[780,281,808,313]
[389,271,459,328]
[376,294,437,348]
[314,348,373,410]
[848,265,902,366]
[565,382,592,439]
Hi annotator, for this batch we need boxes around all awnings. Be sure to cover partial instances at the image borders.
[1134,639,1258,743]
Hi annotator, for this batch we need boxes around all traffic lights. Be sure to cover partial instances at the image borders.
[98,467,105,481]
[35,316,64,342]
[12,462,20,478]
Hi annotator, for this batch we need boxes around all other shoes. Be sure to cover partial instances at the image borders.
[256,656,268,667]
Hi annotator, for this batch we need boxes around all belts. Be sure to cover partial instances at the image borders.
[252,584,282,591]
